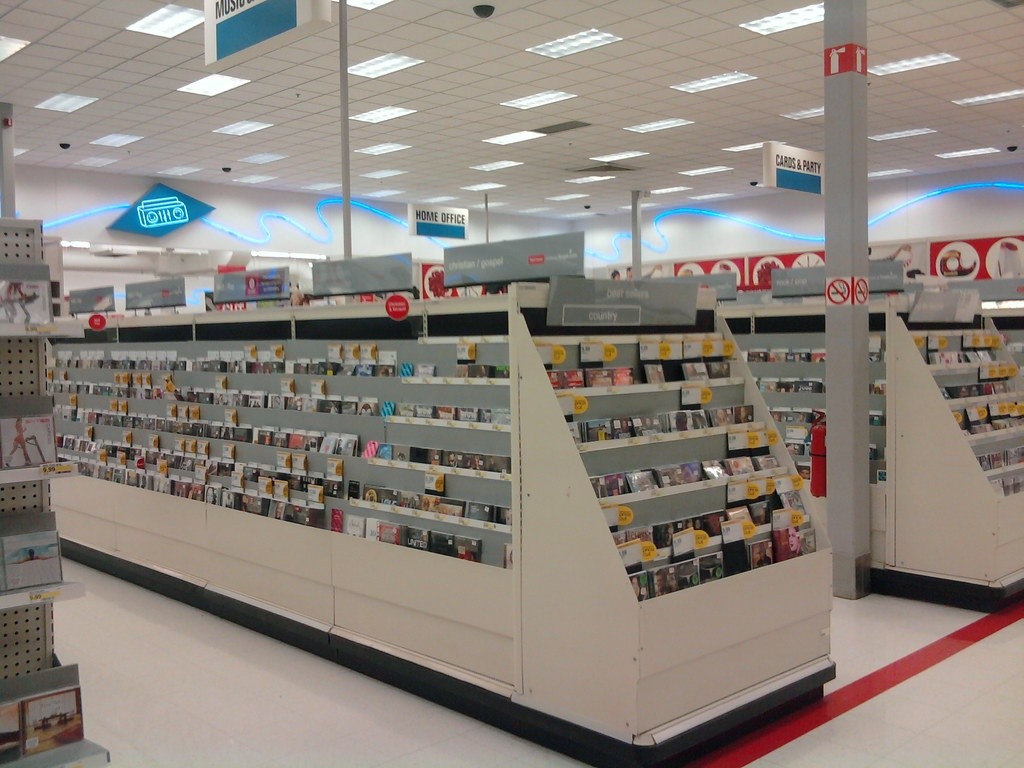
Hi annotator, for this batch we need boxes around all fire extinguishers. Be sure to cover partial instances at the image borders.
[811,409,827,498]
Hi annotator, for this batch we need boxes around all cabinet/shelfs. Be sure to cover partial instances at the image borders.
[730,300,1024,610]
[1,218,113,768]
[44,275,833,768]
